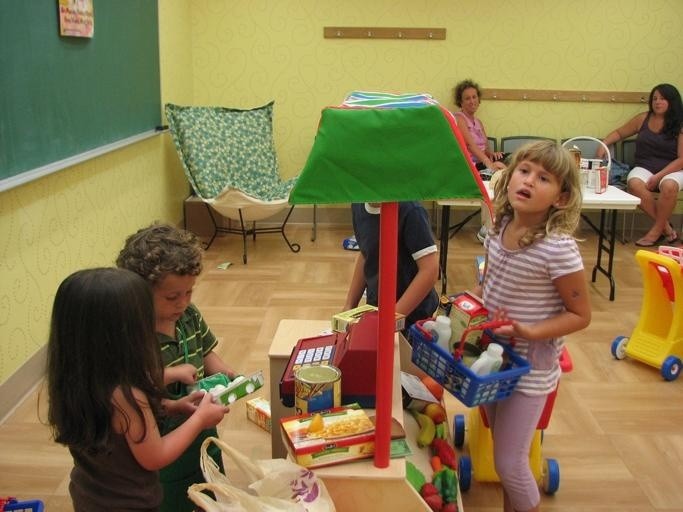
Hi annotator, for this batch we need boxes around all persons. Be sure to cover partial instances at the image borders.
[451,79,506,174]
[41,265,230,511]
[596,84,682,246]
[342,201,443,327]
[464,136,593,512]
[115,222,240,511]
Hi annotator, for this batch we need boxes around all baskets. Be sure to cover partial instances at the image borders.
[410,316,532,408]
[561,136,611,189]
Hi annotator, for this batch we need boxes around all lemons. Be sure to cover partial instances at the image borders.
[307,412,325,434]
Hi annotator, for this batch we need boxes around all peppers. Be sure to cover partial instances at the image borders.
[432,468,458,502]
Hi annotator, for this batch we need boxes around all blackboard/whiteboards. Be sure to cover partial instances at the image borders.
[0,0,163,193]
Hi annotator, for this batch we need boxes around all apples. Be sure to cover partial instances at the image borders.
[424,404,445,425]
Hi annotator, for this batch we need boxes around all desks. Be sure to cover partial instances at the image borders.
[433,180,643,304]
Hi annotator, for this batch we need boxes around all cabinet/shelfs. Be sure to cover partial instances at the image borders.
[283,331,465,512]
[266,318,334,461]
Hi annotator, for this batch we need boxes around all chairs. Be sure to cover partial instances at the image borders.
[486,136,497,154]
[162,98,300,265]
[498,135,558,159]
[560,134,617,233]
[619,136,637,245]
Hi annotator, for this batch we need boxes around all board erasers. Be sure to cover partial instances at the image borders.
[156,126,168,130]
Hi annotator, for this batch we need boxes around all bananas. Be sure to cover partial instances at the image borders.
[410,410,436,449]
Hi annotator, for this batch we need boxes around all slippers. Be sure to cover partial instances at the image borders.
[635,223,680,247]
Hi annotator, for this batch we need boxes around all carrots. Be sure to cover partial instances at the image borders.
[431,449,440,473]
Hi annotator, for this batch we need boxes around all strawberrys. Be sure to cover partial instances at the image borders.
[421,483,456,512]
